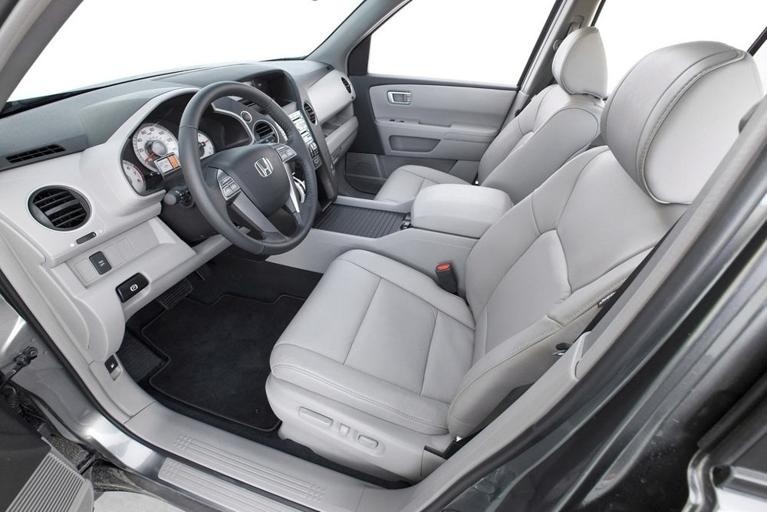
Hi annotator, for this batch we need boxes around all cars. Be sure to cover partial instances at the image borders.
[0,1,767,511]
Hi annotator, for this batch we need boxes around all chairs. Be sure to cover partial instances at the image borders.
[311,27,608,235]
[266,41,764,485]
